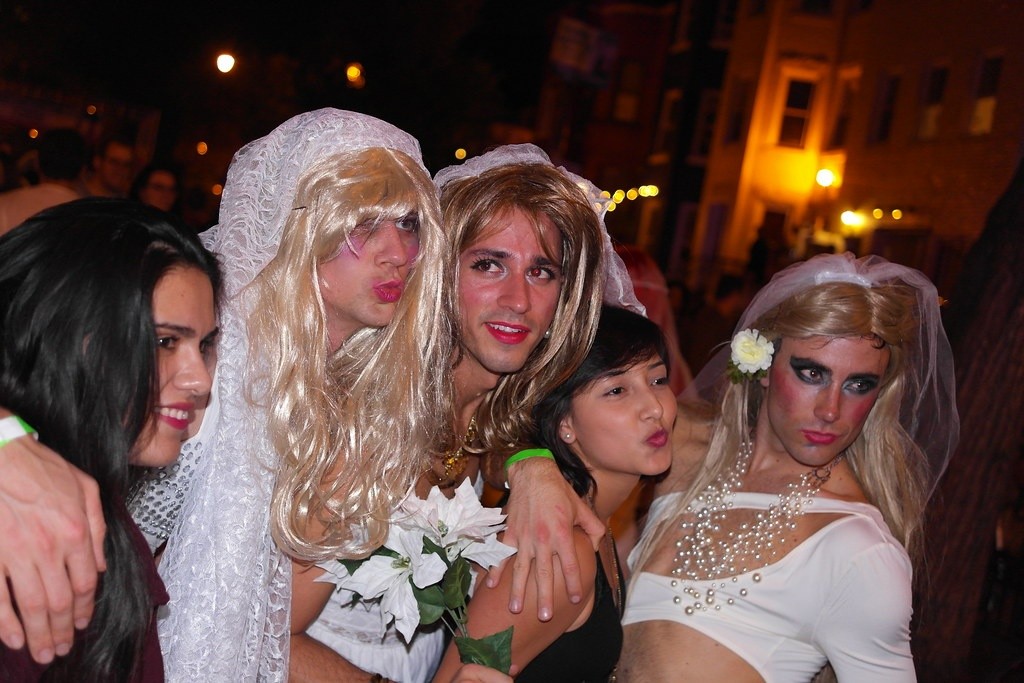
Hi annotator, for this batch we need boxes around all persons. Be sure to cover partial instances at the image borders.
[431,300,680,683]
[0,194,226,682]
[1,107,467,681]
[650,229,799,364]
[431,139,651,683]
[0,122,221,236]
[482,251,961,682]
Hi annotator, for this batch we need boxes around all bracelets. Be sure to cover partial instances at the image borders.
[503,448,556,489]
[2,411,40,451]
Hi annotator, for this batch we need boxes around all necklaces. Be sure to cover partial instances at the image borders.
[670,432,847,617]
[437,404,480,484]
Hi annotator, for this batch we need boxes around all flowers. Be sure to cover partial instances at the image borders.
[726,327,783,429]
[311,471,520,678]
